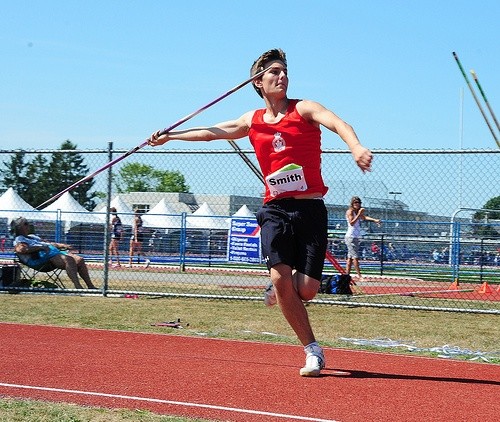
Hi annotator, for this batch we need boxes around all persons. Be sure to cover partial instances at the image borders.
[329,237,404,262]
[126,208,150,269]
[147,49,373,374]
[345,196,381,280]
[109,206,121,268]
[9,217,99,289]
[432,247,500,267]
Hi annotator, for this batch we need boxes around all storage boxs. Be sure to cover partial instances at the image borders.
[0,266,21,288]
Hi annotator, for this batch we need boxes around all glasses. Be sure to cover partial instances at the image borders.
[353,201,362,204]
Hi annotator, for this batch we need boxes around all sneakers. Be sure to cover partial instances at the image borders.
[264,269,298,307]
[300,345,326,376]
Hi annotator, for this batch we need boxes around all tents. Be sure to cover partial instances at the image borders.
[0,187,256,233]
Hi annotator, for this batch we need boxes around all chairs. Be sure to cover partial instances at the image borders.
[13,251,66,289]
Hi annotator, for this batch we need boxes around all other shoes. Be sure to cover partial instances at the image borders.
[126,265,132,268]
[143,259,151,269]
[108,262,112,266]
[113,265,121,268]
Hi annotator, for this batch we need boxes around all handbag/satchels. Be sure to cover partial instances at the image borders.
[319,274,353,295]
